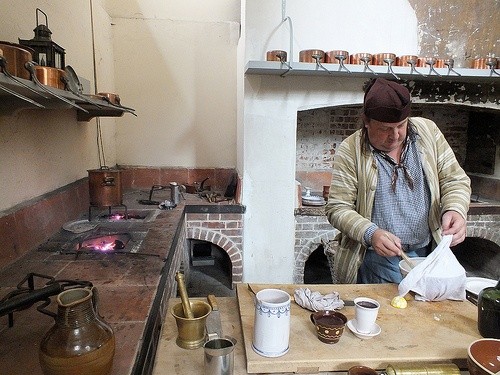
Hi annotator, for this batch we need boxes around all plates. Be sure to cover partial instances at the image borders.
[347,319,381,340]
[62,219,100,233]
[302,195,325,206]
[466,276,498,295]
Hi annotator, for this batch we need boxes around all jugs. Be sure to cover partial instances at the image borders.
[38,285,115,375]
[203,332,235,375]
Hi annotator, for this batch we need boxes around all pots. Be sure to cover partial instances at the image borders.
[0,40,137,117]
[465,279,500,339]
[300,49,463,79]
[266,49,293,69]
[473,57,500,75]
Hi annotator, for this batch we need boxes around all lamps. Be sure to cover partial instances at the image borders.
[18,8,67,70]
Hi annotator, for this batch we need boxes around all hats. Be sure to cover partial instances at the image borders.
[363,78,411,123]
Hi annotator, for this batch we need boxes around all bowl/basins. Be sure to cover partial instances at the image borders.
[399,257,427,296]
[467,338,500,375]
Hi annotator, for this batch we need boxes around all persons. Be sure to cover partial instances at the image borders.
[325,78,470,284]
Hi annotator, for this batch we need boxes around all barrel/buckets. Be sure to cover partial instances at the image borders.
[86,165,126,207]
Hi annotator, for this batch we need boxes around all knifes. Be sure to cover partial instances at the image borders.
[205,295,223,339]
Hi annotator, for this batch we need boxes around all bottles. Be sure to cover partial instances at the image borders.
[380,362,460,375]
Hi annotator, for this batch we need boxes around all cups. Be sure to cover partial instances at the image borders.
[171,301,212,349]
[353,298,380,333]
[251,289,291,358]
[310,310,347,344]
[347,365,377,375]
